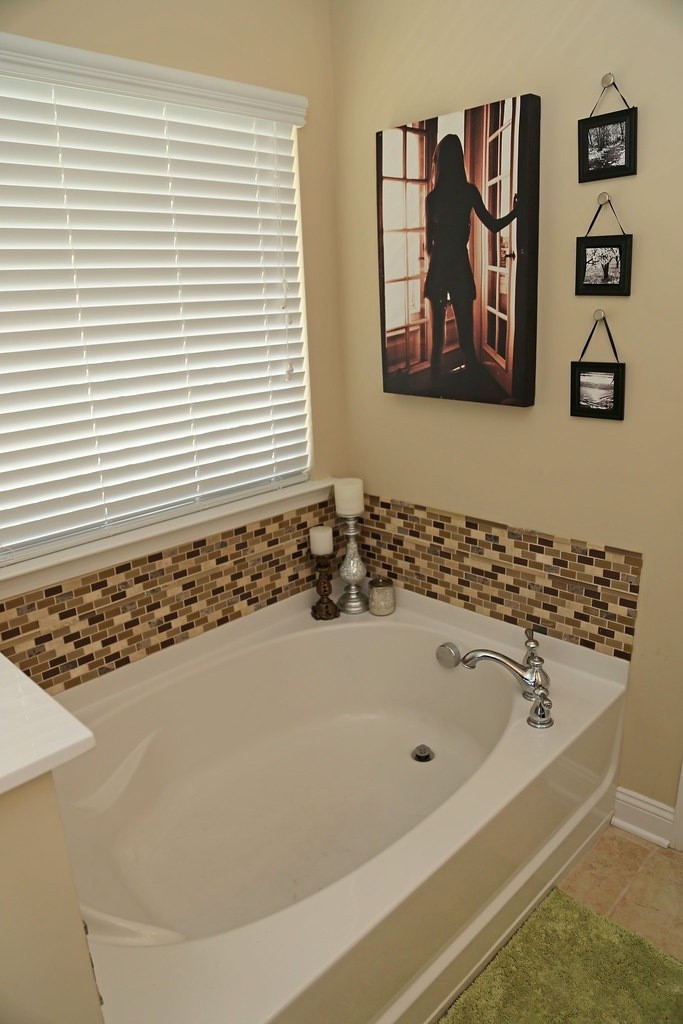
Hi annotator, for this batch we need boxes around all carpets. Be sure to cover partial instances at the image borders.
[435,884,683,1024]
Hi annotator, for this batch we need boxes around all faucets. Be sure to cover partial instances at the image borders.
[459,649,552,702]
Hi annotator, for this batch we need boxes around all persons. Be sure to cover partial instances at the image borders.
[601,255,608,279]
[423,133,521,390]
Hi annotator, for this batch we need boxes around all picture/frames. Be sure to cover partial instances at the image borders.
[570,361,626,421]
[575,234,633,296]
[577,106,639,184]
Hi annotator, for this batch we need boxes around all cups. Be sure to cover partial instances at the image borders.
[369,577,396,615]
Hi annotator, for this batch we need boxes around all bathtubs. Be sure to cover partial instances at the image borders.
[33,570,628,1024]
